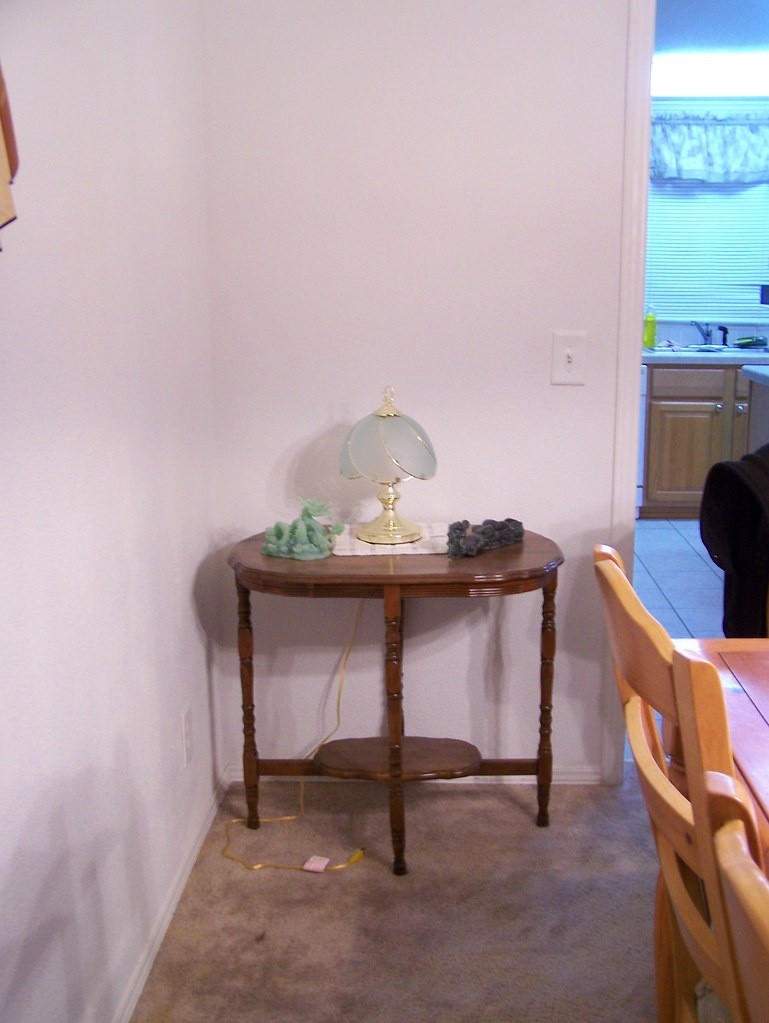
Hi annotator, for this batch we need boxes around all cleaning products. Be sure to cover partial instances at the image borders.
[643,303,657,349]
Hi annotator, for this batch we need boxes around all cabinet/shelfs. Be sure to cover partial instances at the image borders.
[644,366,749,518]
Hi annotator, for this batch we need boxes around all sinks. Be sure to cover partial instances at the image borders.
[715,346,769,354]
[643,347,721,354]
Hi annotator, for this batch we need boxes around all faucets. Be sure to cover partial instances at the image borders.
[690,320,713,345]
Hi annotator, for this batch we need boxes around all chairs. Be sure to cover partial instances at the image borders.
[591,543,769,1023]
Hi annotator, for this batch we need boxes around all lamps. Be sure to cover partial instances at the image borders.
[338,386,439,544]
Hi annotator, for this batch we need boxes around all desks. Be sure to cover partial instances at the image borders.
[663,638,768,850]
[227,523,565,877]
